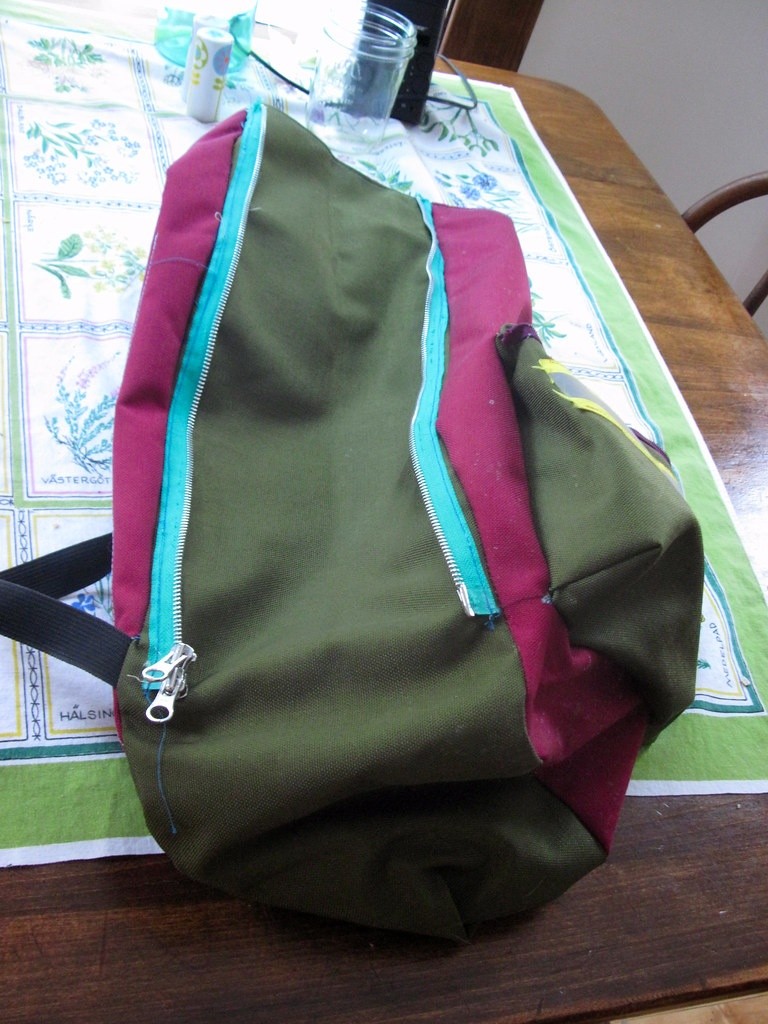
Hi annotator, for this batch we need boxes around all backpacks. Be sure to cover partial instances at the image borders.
[3,103,702,945]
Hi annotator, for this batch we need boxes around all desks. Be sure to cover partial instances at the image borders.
[0,0,768,1024]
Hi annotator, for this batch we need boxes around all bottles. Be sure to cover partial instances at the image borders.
[306,0,417,156]
